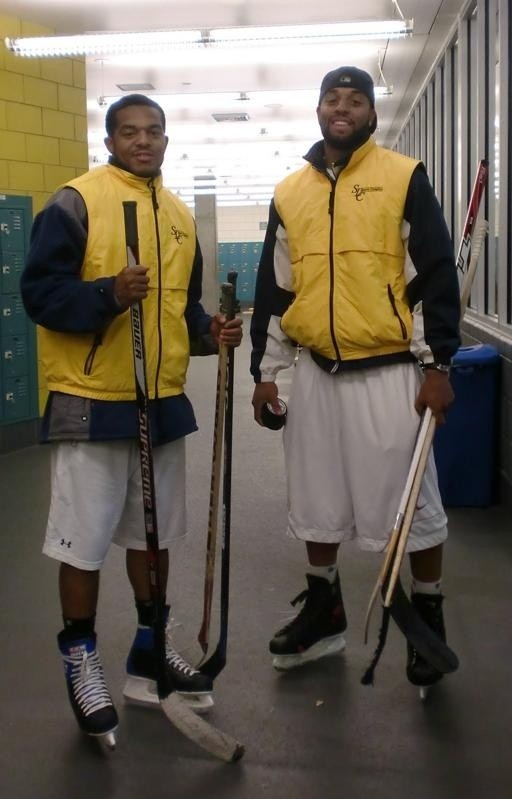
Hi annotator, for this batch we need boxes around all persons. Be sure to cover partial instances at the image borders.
[19,92,243,739]
[248,65,463,688]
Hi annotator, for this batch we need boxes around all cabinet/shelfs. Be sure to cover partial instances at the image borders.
[0,192,38,426]
[217,241,265,309]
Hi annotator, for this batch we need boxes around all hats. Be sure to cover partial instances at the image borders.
[317,65,378,136]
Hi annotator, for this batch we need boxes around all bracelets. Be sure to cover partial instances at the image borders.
[420,362,450,375]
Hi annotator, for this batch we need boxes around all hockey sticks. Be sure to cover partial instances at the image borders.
[361,216,490,685]
[363,158,492,685]
[200,270,239,685]
[185,284,231,668]
[122,201,244,765]
[124,200,244,763]
[361,159,490,644]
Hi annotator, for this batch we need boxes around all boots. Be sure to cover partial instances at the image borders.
[56,628,120,739]
[125,602,215,693]
[269,571,351,657]
[405,589,446,688]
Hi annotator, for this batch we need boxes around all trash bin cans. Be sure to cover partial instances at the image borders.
[432,343,500,508]
